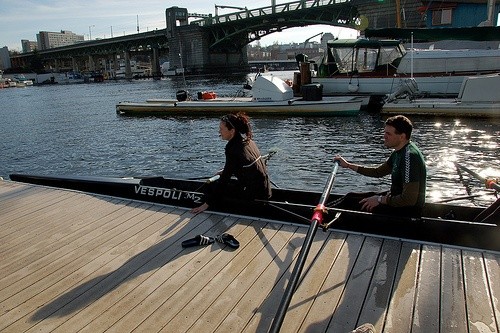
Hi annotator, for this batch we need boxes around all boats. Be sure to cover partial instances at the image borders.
[241,32,500,98]
[11,172,500,256]
[116,74,363,117]
[381,74,500,117]
[0,70,33,89]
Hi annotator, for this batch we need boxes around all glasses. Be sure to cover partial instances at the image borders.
[223,115,235,128]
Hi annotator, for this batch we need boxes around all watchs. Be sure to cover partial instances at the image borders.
[377,195,383,205]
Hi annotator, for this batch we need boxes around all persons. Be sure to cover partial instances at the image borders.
[309,115,427,239]
[473,198,500,224]
[190,112,272,215]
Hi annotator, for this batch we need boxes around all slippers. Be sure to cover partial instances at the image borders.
[182,234,215,248]
[215,233,239,249]
